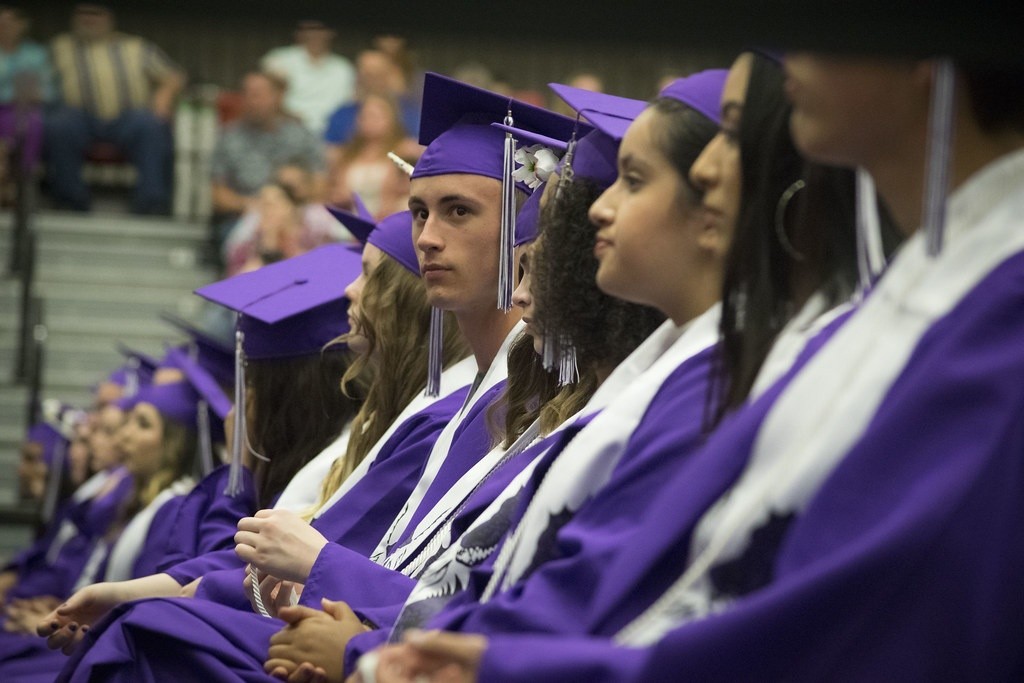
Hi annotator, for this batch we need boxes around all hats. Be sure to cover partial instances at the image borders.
[410,69,733,387]
[109,315,235,420]
[193,190,444,498]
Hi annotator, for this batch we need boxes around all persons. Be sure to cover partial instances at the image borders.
[0,51,1024,682]
[0,1,604,276]
